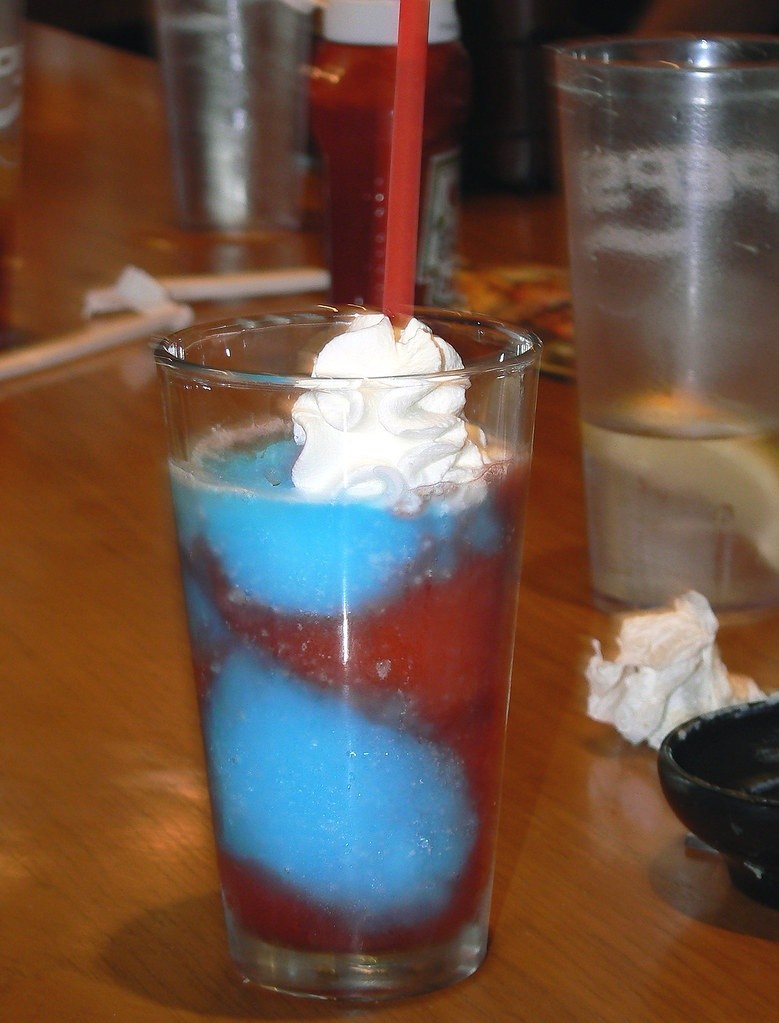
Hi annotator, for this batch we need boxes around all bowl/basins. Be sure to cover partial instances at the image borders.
[658,699,779,911]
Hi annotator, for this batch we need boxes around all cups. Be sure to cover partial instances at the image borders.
[162,0,311,228]
[556,36,779,621]
[154,305,543,1000]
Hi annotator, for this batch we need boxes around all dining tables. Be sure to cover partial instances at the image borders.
[2,12,779,1023]
